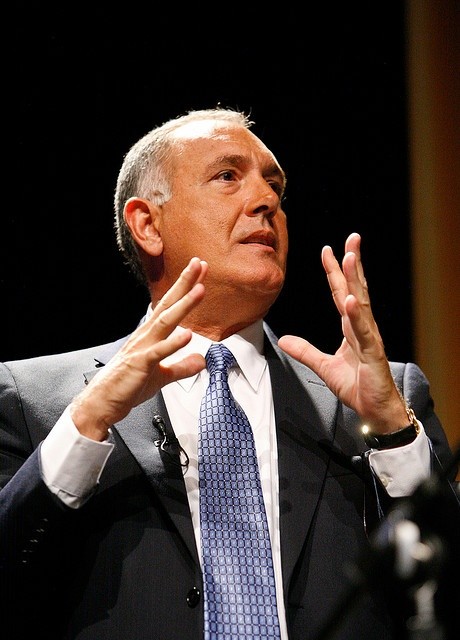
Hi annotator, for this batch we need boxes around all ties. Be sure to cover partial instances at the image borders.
[198,345,281,640]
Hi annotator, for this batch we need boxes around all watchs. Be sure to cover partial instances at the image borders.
[360,408,420,450]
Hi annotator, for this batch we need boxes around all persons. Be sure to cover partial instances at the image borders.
[0,104,460,640]
